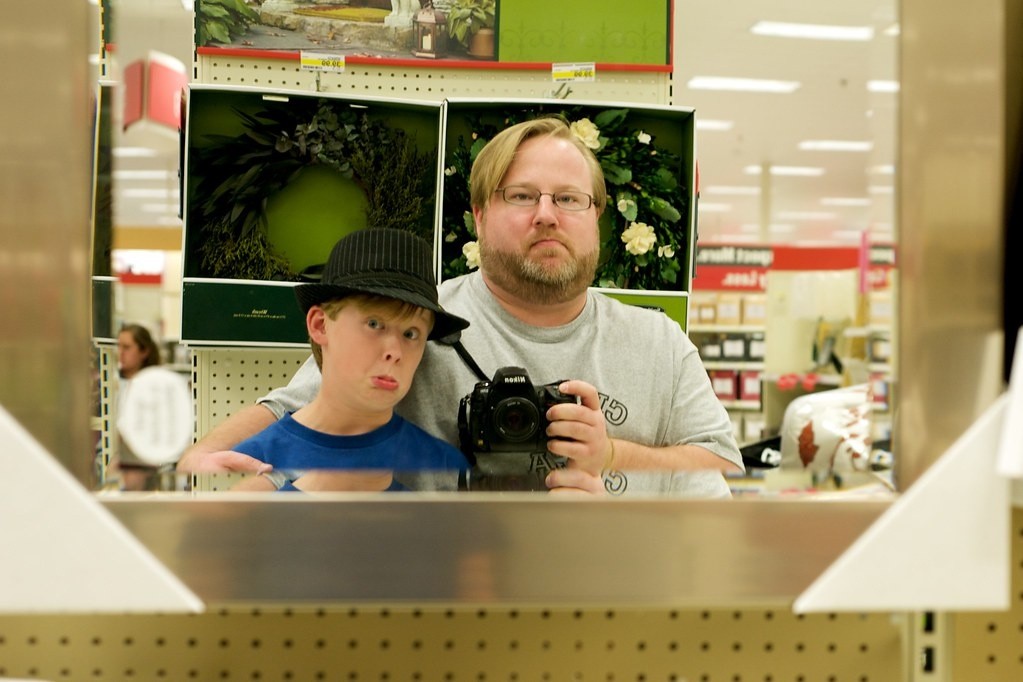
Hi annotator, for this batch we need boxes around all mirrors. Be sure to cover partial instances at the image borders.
[0,0,1023,612]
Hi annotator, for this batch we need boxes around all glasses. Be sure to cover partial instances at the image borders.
[495,184,600,212]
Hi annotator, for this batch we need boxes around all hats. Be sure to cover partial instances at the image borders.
[295,228,471,341]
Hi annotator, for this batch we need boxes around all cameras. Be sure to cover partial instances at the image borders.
[458,366,577,453]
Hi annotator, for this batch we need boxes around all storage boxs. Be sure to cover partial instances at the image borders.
[689,298,765,400]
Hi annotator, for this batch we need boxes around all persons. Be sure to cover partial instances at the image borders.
[114,326,162,471]
[229,227,470,470]
[178,118,743,472]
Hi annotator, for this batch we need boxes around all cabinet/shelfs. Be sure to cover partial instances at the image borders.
[687,289,770,411]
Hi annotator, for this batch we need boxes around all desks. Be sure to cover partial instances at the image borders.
[759,367,840,387]
